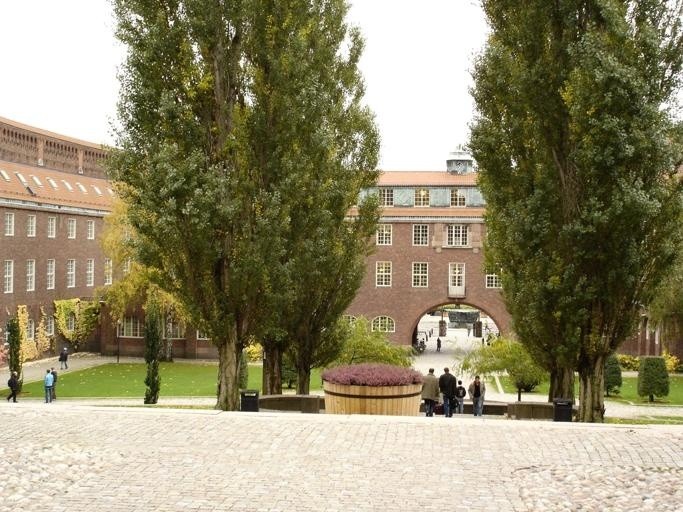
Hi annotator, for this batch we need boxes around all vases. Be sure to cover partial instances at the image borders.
[324,379,423,414]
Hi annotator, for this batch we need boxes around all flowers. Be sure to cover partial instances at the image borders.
[323,361,423,385]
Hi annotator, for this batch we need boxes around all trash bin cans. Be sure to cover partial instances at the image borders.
[240,390,259,412]
[552,398,573,422]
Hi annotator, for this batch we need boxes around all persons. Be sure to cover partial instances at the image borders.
[44,366,57,404]
[58,347,68,370]
[420,367,486,417]
[7,371,19,403]
[436,337,442,351]
